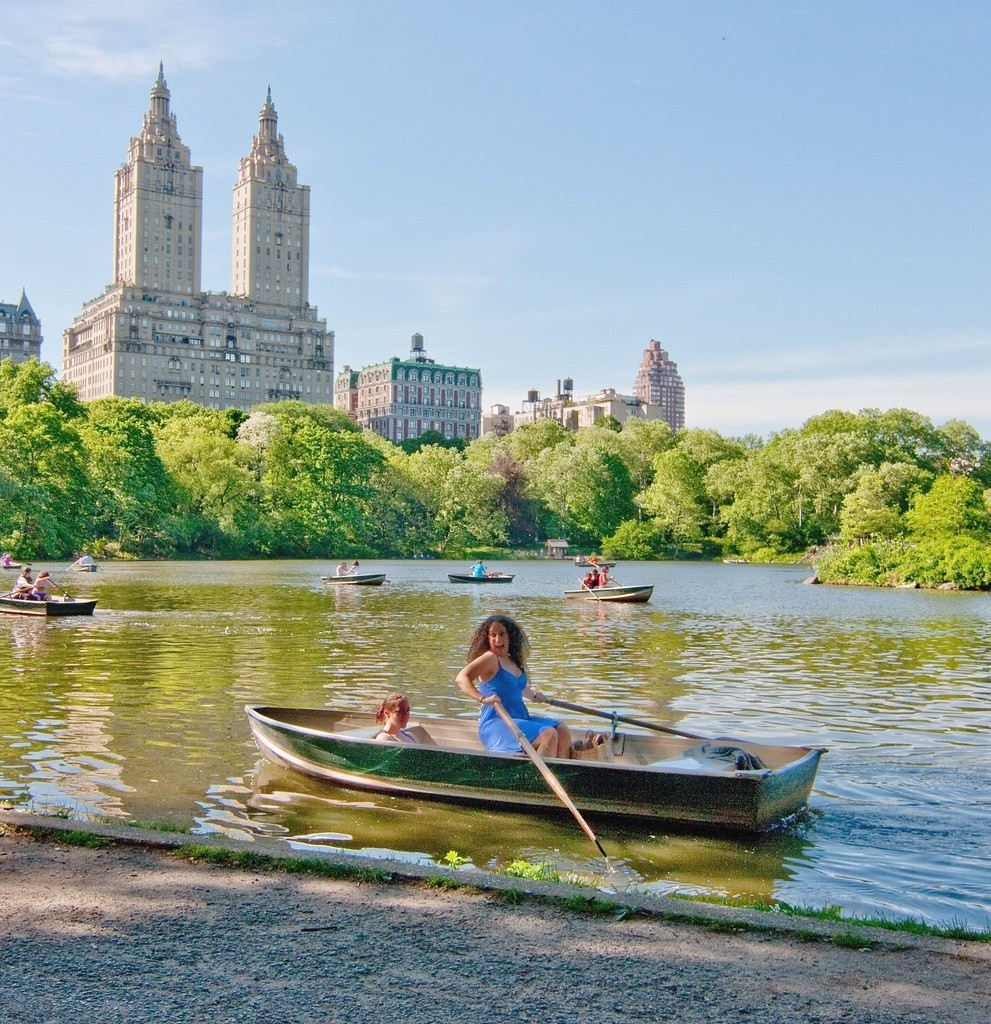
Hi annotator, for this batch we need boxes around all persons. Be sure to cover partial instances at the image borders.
[336,561,349,576]
[375,693,420,744]
[1,553,11,567]
[11,566,37,600]
[591,556,597,565]
[32,570,58,601]
[598,566,614,586]
[541,547,564,559]
[455,616,571,760]
[581,569,600,590]
[346,561,360,576]
[576,554,587,562]
[78,553,93,566]
[471,561,487,577]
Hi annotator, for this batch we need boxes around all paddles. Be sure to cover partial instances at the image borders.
[48,578,72,598]
[1,585,35,599]
[92,560,104,571]
[65,558,81,572]
[587,560,623,586]
[534,694,756,744]
[577,577,602,602]
[494,702,610,860]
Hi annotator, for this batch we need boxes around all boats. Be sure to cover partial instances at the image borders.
[0,590,99,617]
[71,564,97,572]
[321,573,386,586]
[244,705,830,830]
[447,573,516,583]
[723,560,751,565]
[564,584,655,604]
[0,561,22,569]
[576,562,616,567]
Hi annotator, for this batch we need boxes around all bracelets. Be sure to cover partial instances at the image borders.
[480,696,485,704]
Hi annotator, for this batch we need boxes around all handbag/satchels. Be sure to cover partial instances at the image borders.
[570,733,615,762]
